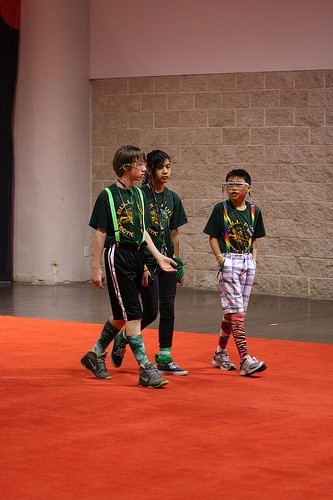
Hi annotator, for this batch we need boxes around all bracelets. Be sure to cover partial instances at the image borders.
[144,264,148,271]
[157,255,164,263]
[215,255,223,262]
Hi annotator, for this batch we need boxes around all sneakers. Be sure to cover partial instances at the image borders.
[110,335,128,368]
[211,350,236,371]
[80,351,113,379]
[155,354,188,376]
[239,356,267,375]
[138,361,169,388]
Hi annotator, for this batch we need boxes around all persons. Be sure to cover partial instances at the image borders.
[80,146,177,389]
[203,169,267,376]
[109,149,189,376]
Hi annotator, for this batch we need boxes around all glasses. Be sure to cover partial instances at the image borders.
[223,182,249,190]
[118,162,147,171]
